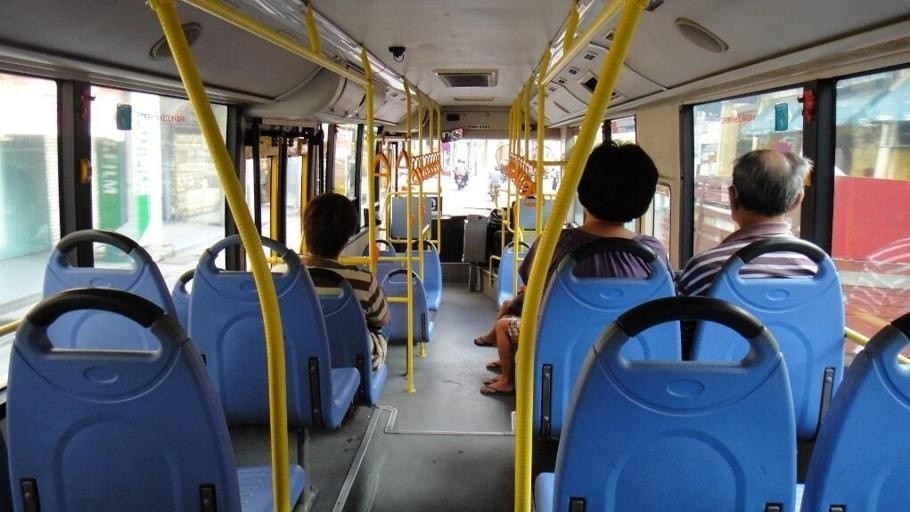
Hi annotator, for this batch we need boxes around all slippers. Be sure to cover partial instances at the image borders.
[486,360,501,369]
[482,376,501,384]
[471,336,499,347]
[480,386,515,396]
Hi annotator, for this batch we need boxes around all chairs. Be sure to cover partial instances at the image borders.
[498,196,910,512]
[0,227,441,512]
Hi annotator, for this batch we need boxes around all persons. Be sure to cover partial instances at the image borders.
[269,192,391,372]
[676,149,820,358]
[487,164,503,193]
[480,314,525,395]
[473,285,525,369]
[453,157,467,177]
[519,139,677,307]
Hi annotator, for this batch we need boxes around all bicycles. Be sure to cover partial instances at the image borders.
[489,175,500,201]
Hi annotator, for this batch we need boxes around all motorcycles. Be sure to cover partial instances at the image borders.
[453,168,472,190]
